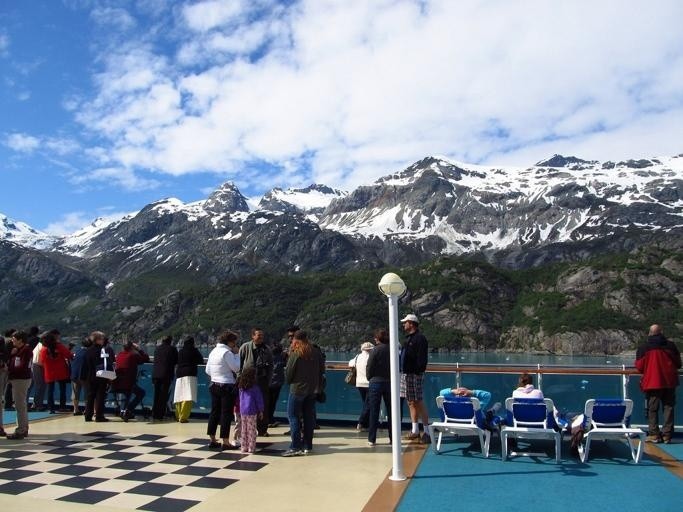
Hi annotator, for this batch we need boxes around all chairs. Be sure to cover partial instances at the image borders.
[105,366,144,421]
[578,399,647,464]
[501,397,562,463]
[428,396,491,458]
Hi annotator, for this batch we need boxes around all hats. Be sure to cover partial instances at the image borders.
[401,314,420,324]
[294,329,307,339]
[361,342,374,350]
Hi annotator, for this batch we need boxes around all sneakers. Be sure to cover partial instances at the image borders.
[280,447,309,456]
[209,431,269,456]
[1,426,28,440]
[367,441,375,445]
[5,403,111,422]
[356,425,363,430]
[402,432,421,438]
[645,435,672,444]
[422,433,431,444]
[119,409,190,422]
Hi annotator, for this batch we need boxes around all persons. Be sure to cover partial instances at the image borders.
[204,326,325,457]
[349,341,375,432]
[440,386,502,419]
[635,324,681,443]
[512,374,545,401]
[366,328,394,446]
[0,326,204,440]
[400,314,431,445]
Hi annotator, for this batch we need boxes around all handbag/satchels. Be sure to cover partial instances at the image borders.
[344,371,356,384]
[96,353,117,380]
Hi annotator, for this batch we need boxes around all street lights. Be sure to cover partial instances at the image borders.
[377,272,408,483]
